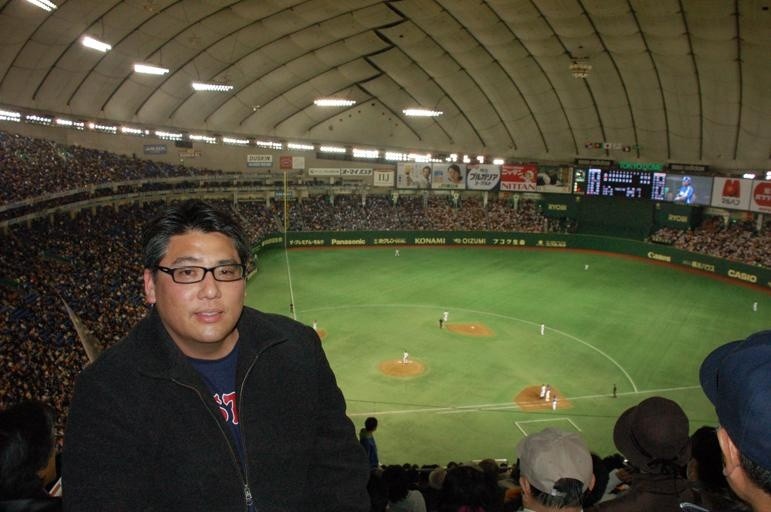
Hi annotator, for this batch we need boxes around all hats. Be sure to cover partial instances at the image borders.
[428,467,447,490]
[698,328,771,472]
[515,425,596,498]
[612,394,693,475]
[479,457,502,481]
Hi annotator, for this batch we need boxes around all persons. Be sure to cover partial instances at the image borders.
[443,310,449,321]
[288,160,770,271]
[752,299,759,312]
[541,323,545,336]
[313,319,318,331]
[613,383,617,398]
[1,132,286,489]
[360,416,742,512]
[541,383,558,411]
[588,395,702,512]
[0,396,64,512]
[403,350,409,363]
[438,318,444,328]
[699,326,771,512]
[58,195,375,510]
[289,302,294,315]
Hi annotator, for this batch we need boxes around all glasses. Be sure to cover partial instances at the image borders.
[156,260,249,286]
